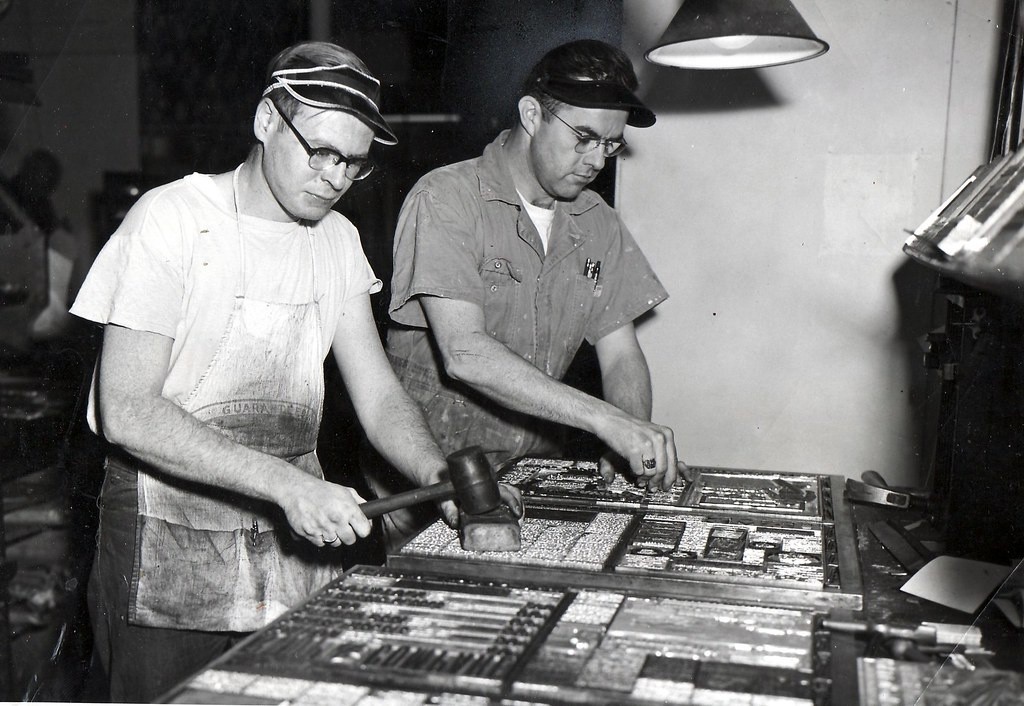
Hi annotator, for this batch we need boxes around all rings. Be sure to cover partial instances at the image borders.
[643,459,656,469]
[322,536,337,543]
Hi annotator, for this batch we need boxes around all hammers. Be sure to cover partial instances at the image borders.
[276,444,503,545]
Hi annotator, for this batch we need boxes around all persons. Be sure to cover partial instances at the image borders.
[67,41,522,704]
[369,40,693,557]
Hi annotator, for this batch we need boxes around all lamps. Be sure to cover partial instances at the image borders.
[644,0,831,68]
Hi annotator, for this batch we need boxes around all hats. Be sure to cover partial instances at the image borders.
[523,77,657,128]
[262,64,399,146]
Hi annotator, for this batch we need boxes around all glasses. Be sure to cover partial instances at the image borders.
[536,97,628,157]
[267,94,375,181]
